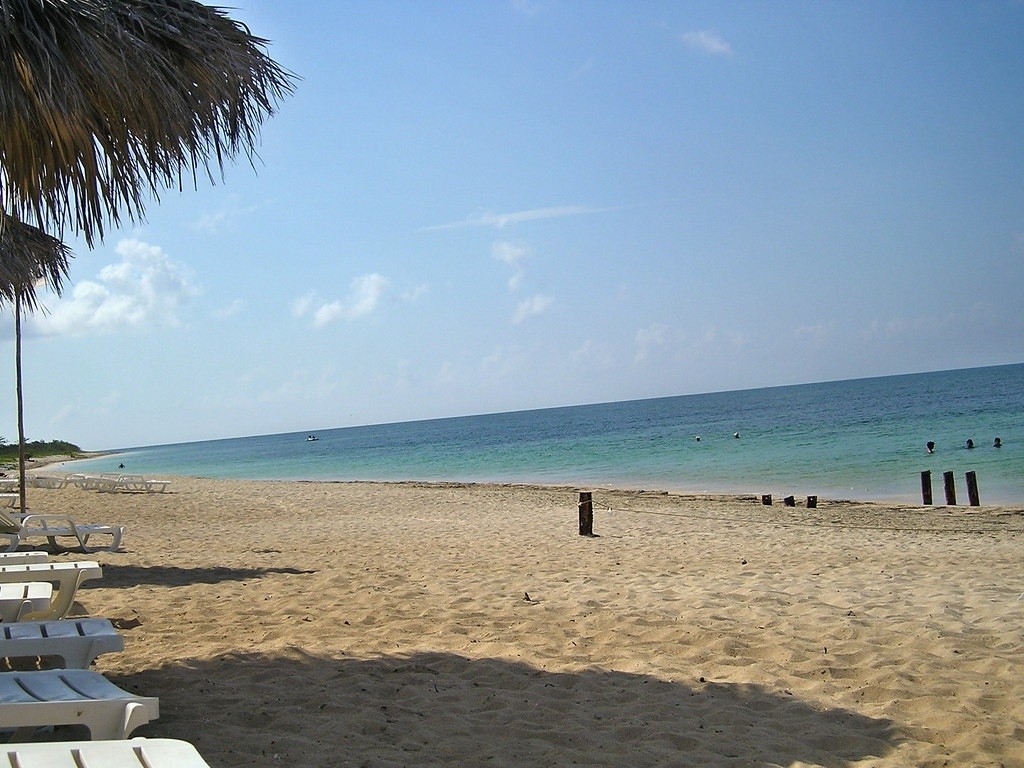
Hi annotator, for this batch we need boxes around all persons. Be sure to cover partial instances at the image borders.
[927,442,935,453]
[966,439,975,449]
[993,438,1002,448]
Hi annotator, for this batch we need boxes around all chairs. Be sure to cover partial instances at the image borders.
[0,473,212,768]
